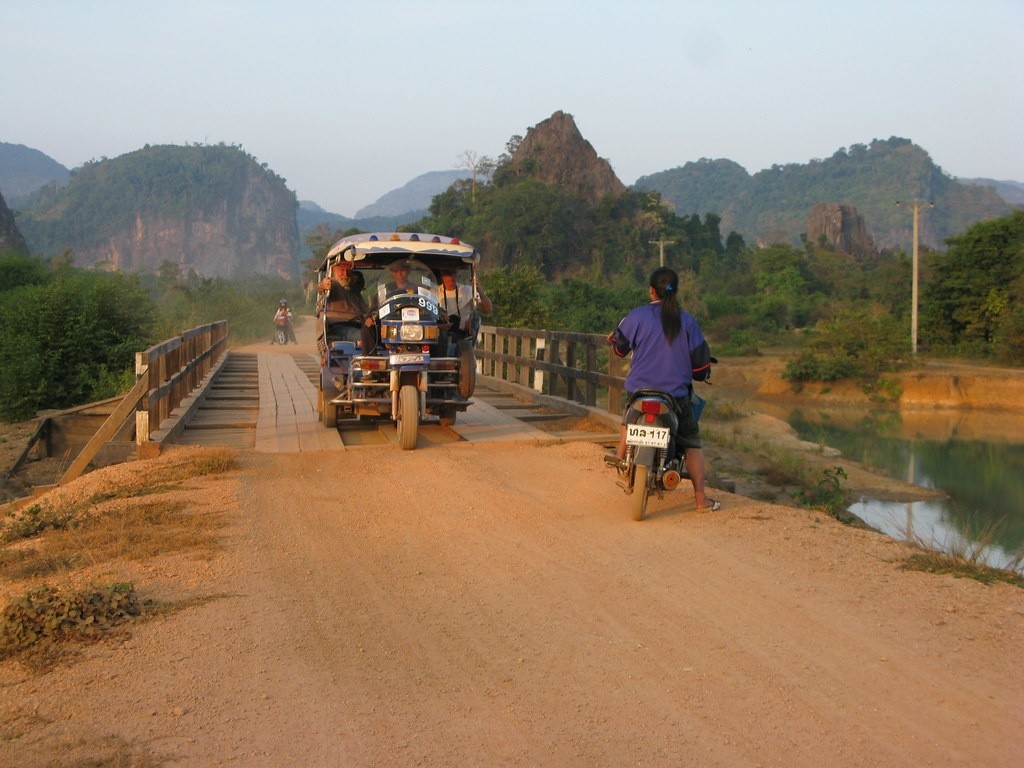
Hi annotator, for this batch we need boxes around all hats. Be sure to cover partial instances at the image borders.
[390,260,410,271]
[440,264,456,275]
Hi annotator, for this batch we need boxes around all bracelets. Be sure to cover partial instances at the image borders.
[480,293,486,298]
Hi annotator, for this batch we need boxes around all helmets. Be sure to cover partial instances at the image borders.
[280,299,287,303]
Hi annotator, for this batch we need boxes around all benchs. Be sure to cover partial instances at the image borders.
[328,340,357,354]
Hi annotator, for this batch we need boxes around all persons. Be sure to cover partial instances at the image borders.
[317,259,383,350]
[607,267,721,513]
[429,261,493,398]
[270,299,298,345]
[360,256,442,382]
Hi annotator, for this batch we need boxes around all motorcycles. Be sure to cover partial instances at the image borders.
[317,231,481,452]
[604,357,717,521]
[274,309,293,344]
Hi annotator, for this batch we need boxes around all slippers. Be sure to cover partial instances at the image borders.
[697,498,720,512]
